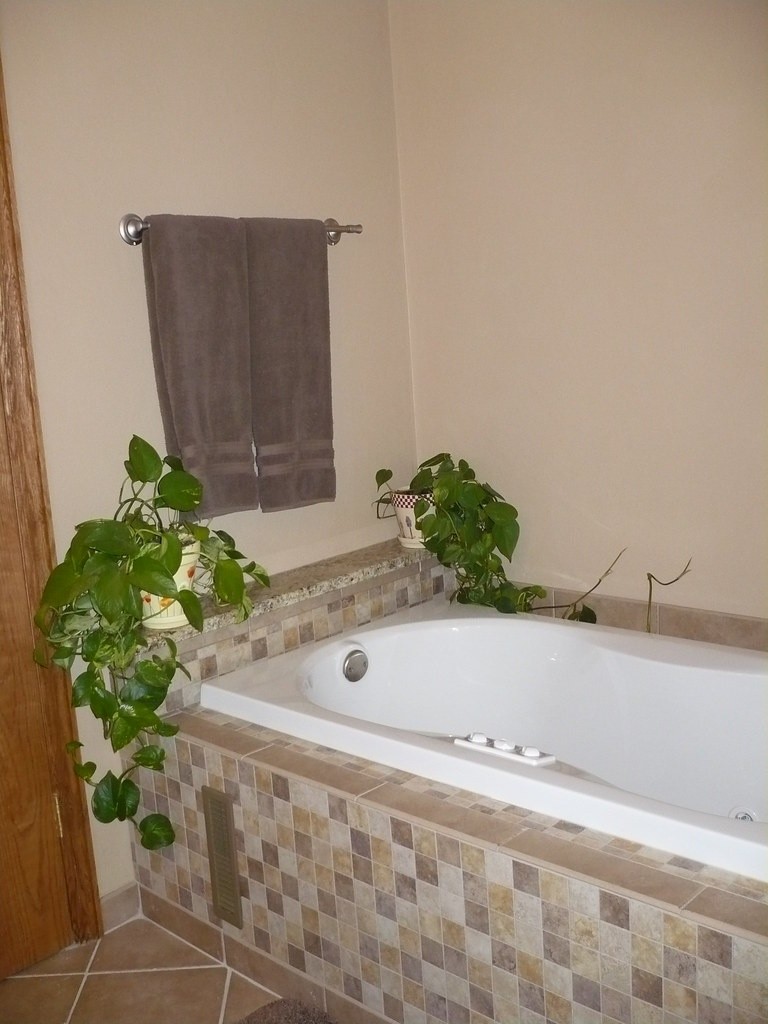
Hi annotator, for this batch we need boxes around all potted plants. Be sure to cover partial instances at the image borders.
[39,435,270,851]
[372,452,693,631]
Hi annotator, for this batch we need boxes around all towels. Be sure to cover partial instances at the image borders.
[142,213,337,520]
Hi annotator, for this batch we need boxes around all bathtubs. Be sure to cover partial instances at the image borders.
[105,534,768,1023]
